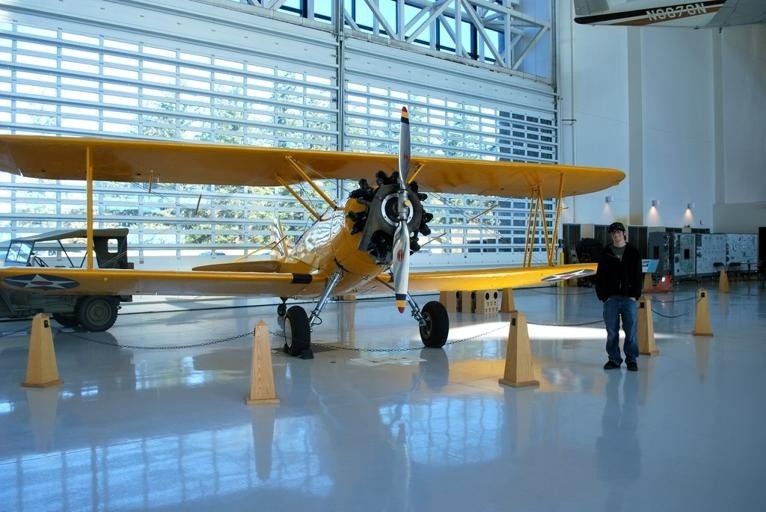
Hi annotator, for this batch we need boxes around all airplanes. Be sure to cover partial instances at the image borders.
[0,107,627,356]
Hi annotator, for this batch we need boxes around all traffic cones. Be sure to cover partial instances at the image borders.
[498,288,518,313]
[246,320,280,406]
[718,266,730,293]
[20,313,64,388]
[638,297,661,355]
[497,311,539,387]
[641,272,655,294]
[692,287,712,337]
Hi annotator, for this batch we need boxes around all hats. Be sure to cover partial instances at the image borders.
[609,223,625,233]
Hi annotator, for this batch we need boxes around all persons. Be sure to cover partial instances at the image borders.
[594,222,643,372]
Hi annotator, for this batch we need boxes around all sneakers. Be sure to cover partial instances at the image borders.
[625,358,637,371]
[605,361,620,369]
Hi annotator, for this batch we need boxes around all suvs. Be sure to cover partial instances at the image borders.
[0,227,133,332]
